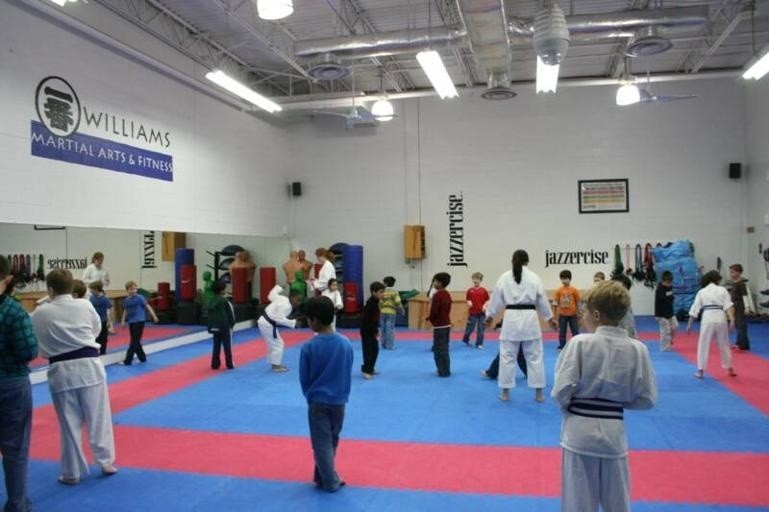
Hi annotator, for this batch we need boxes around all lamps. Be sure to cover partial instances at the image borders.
[533,55,565,98]
[736,0,769,84]
[202,56,286,117]
[413,1,466,103]
[372,70,398,125]
[257,0,294,21]
[615,62,640,107]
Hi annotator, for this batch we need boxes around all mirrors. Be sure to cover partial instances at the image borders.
[0,220,297,378]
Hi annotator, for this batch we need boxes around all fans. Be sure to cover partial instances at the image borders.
[623,56,701,104]
[306,68,400,132]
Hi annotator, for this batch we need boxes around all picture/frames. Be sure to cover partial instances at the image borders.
[576,178,631,215]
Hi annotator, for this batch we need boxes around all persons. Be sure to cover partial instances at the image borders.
[311,248,337,292]
[282,249,312,303]
[0,254,38,512]
[206,272,490,379]
[479,262,751,381]
[203,270,215,306]
[298,296,354,494]
[229,250,257,282]
[482,248,560,403]
[70,251,117,356]
[551,281,656,512]
[118,279,158,365]
[27,269,118,485]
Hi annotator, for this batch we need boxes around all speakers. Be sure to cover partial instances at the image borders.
[729,163,740,178]
[293,183,300,195]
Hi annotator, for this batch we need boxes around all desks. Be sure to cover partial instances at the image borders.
[14,289,128,330]
[405,290,589,335]
